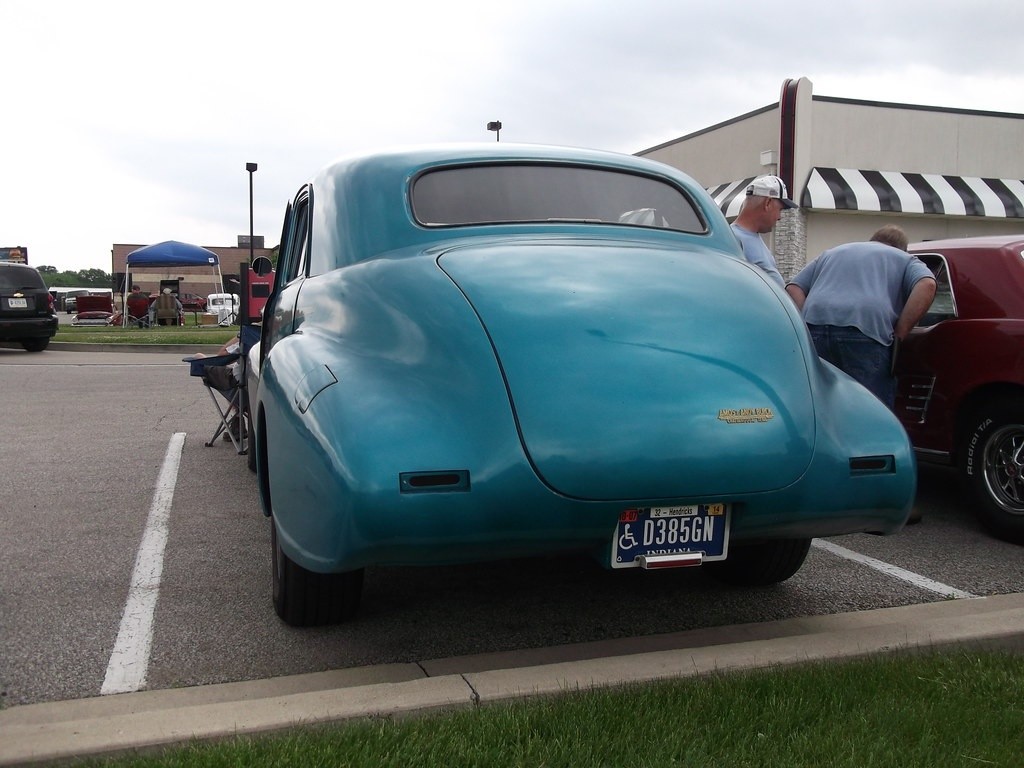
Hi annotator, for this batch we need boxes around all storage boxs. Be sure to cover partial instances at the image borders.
[202,314,218,324]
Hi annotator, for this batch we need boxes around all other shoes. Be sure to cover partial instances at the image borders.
[906,508,922,524]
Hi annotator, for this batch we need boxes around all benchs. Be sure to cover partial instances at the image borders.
[72,294,118,322]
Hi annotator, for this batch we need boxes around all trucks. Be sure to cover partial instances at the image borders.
[61,290,92,314]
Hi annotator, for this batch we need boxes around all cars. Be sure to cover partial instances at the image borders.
[887,232,1024,545]
[178,292,207,311]
[205,294,241,324]
[241,140,921,626]
[70,294,122,328]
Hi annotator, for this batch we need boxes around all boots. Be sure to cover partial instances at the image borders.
[223,417,248,441]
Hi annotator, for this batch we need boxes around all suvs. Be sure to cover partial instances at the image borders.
[0,261,59,353]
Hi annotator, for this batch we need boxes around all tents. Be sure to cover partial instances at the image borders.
[122,240,231,328]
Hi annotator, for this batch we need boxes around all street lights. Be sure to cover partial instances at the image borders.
[245,163,258,268]
[487,119,503,142]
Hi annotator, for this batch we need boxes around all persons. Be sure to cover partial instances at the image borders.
[784,224,938,524]
[194,305,265,442]
[729,175,798,289]
[126,285,183,329]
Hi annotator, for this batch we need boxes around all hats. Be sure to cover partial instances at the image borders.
[131,285,140,291]
[163,288,172,294]
[746,175,798,208]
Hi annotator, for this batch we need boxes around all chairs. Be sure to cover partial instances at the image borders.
[127,296,152,329]
[181,324,261,456]
[153,294,181,326]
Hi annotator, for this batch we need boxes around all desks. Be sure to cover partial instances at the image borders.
[183,302,200,326]
